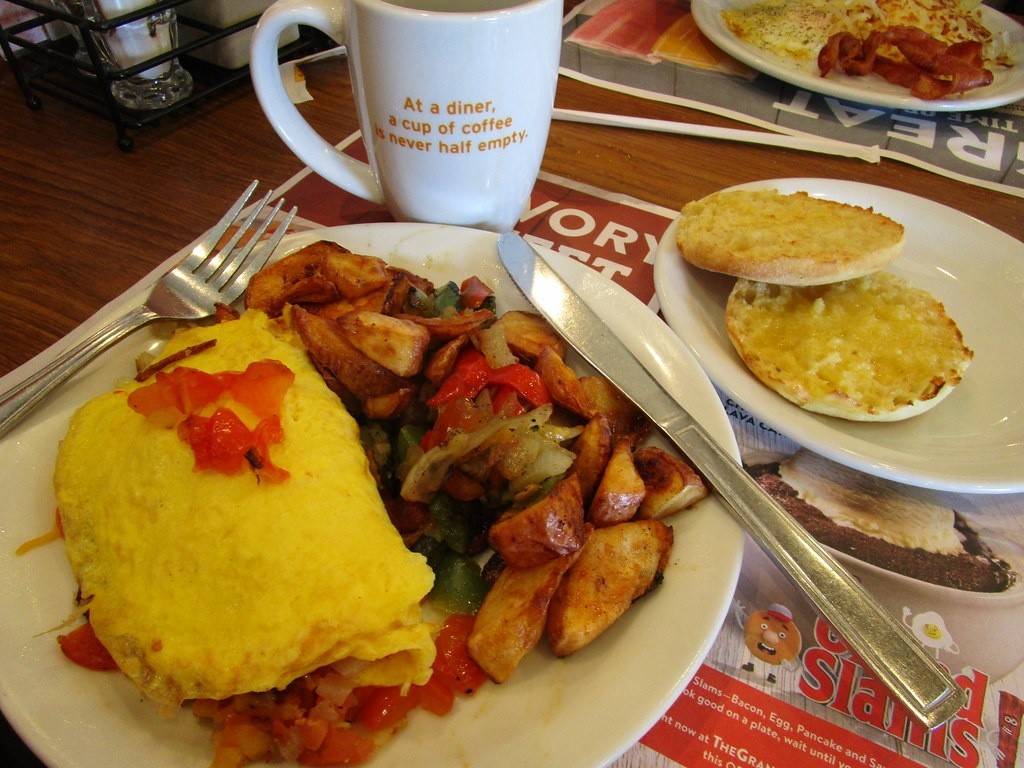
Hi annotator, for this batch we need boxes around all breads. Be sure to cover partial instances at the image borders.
[726,270,973,422]
[677,188,903,286]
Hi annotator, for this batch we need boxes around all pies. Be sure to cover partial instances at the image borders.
[56,312,437,703]
[721,0,1015,79]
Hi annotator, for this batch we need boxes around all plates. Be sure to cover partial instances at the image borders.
[690,0,1024,112]
[0,221,746,768]
[652,176,1024,495]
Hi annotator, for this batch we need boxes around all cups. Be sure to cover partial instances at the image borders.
[247,0,564,235]
[50,0,194,111]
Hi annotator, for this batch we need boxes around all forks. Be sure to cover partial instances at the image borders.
[0,178,299,439]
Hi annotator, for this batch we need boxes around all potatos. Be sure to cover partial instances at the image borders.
[253,243,708,685]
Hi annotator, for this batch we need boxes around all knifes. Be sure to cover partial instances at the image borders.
[496,228,968,734]
